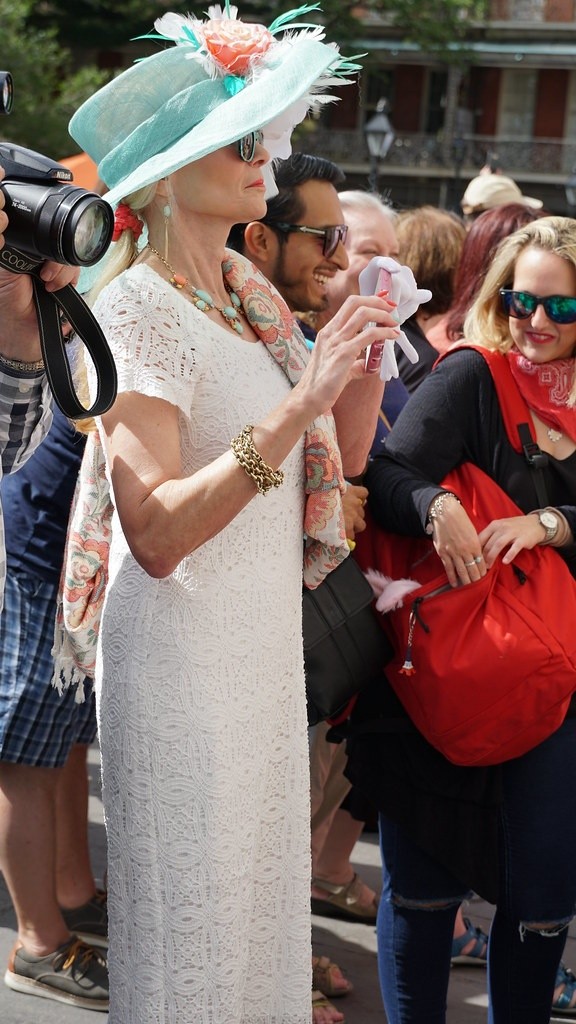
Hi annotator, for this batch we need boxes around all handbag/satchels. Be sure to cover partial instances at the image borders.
[301,554,395,726]
[348,341,576,767]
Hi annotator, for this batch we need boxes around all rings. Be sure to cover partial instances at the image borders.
[475,556,482,563]
[465,561,475,566]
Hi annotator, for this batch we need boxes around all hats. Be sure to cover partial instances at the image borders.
[462,174,543,211]
[67,0,368,211]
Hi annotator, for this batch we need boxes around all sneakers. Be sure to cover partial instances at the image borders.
[3,935,109,1012]
[59,889,108,949]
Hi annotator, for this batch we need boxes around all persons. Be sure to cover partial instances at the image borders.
[0,162,84,476]
[1,334,111,1012]
[69,0,402,1024]
[225,154,576,1024]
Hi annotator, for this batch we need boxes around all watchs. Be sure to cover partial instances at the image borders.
[538,511,558,544]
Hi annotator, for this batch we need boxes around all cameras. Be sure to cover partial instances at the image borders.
[0,72,113,267]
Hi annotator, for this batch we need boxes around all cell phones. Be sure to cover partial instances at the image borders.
[366,268,394,374]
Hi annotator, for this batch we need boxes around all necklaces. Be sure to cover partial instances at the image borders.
[547,429,562,442]
[147,242,246,333]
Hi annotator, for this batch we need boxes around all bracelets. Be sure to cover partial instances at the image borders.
[229,424,286,496]
[425,493,461,535]
[0,353,46,374]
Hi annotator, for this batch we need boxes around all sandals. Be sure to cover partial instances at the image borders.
[312,955,354,998]
[311,872,380,925]
[310,989,346,1024]
[450,915,489,964]
[552,962,576,1013]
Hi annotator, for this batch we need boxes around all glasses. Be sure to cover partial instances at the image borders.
[239,131,264,162]
[499,285,576,324]
[241,220,348,258]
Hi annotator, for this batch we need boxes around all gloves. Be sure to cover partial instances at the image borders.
[359,255,433,381]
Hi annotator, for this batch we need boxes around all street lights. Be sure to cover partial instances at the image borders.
[363,98,396,193]
